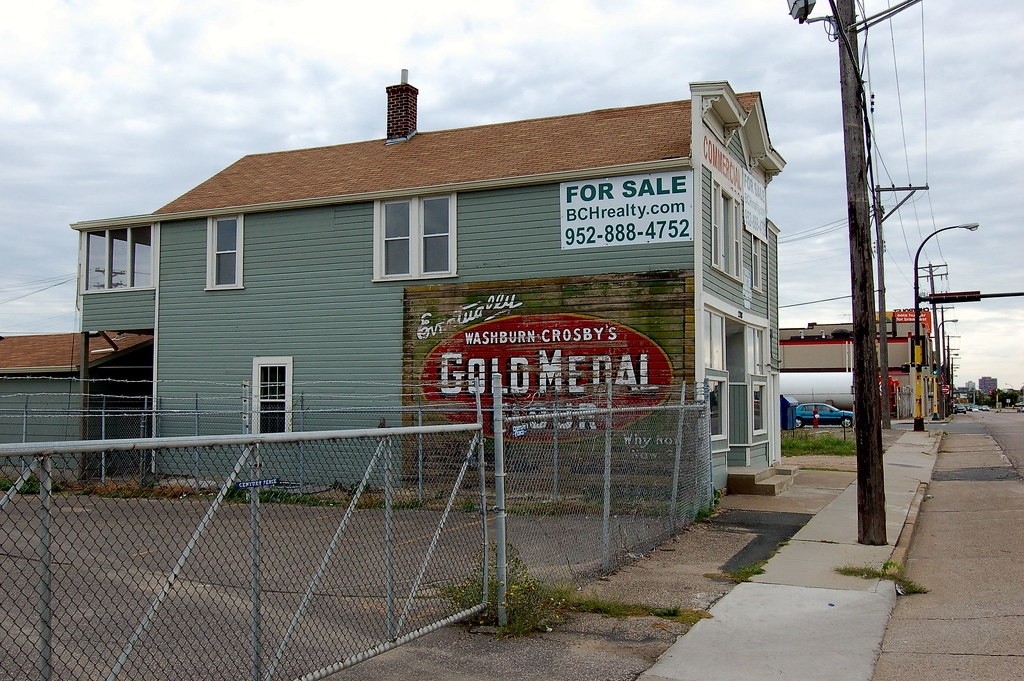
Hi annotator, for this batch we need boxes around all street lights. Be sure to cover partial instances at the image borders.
[1005,383,1013,389]
[938,318,959,408]
[911,222,979,432]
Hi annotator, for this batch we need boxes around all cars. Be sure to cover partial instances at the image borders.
[796,402,854,427]
[981,405,990,411]
[952,403,980,414]
[979,405,983,410]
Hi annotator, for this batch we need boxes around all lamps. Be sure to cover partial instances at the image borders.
[799,330,804,339]
[820,330,825,338]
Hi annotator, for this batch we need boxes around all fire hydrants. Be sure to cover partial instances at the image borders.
[812,409,819,428]
